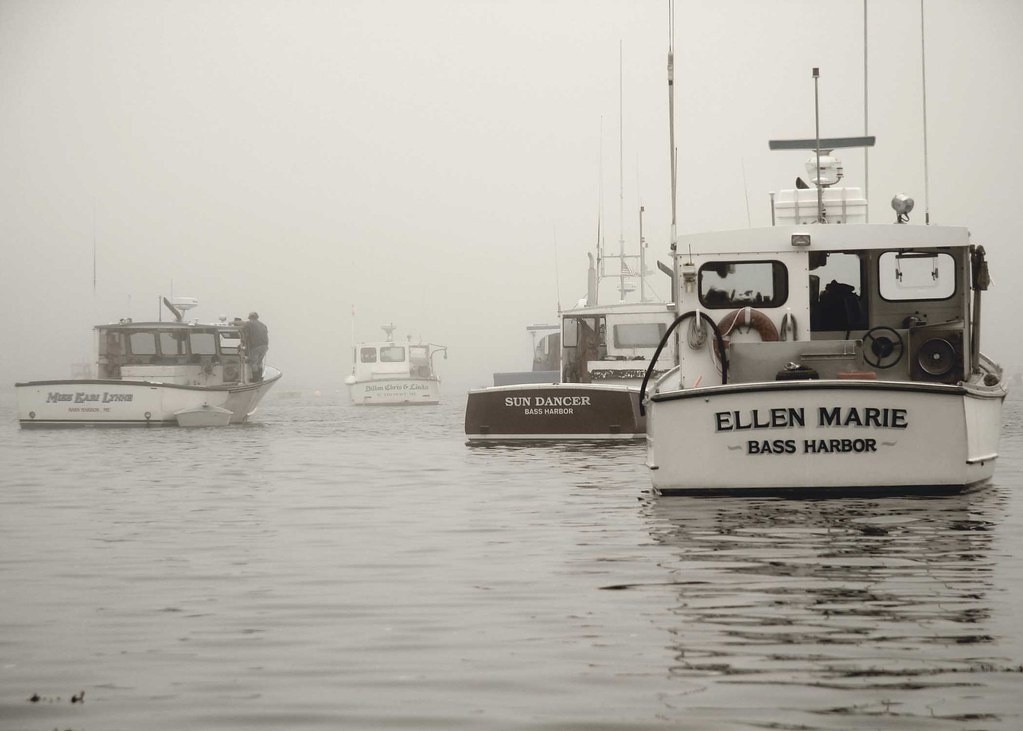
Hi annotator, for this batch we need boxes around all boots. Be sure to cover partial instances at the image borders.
[250,367,263,383]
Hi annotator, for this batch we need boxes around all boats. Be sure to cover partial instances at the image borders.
[460,2,1010,497]
[14,296,283,430]
[343,320,448,407]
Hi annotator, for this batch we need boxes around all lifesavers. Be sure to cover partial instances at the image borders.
[715,309,779,367]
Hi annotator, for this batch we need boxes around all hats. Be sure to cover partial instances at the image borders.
[248,312,259,319]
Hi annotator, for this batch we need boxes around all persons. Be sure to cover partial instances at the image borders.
[106,334,121,380]
[229,311,269,382]
[564,344,585,383]
